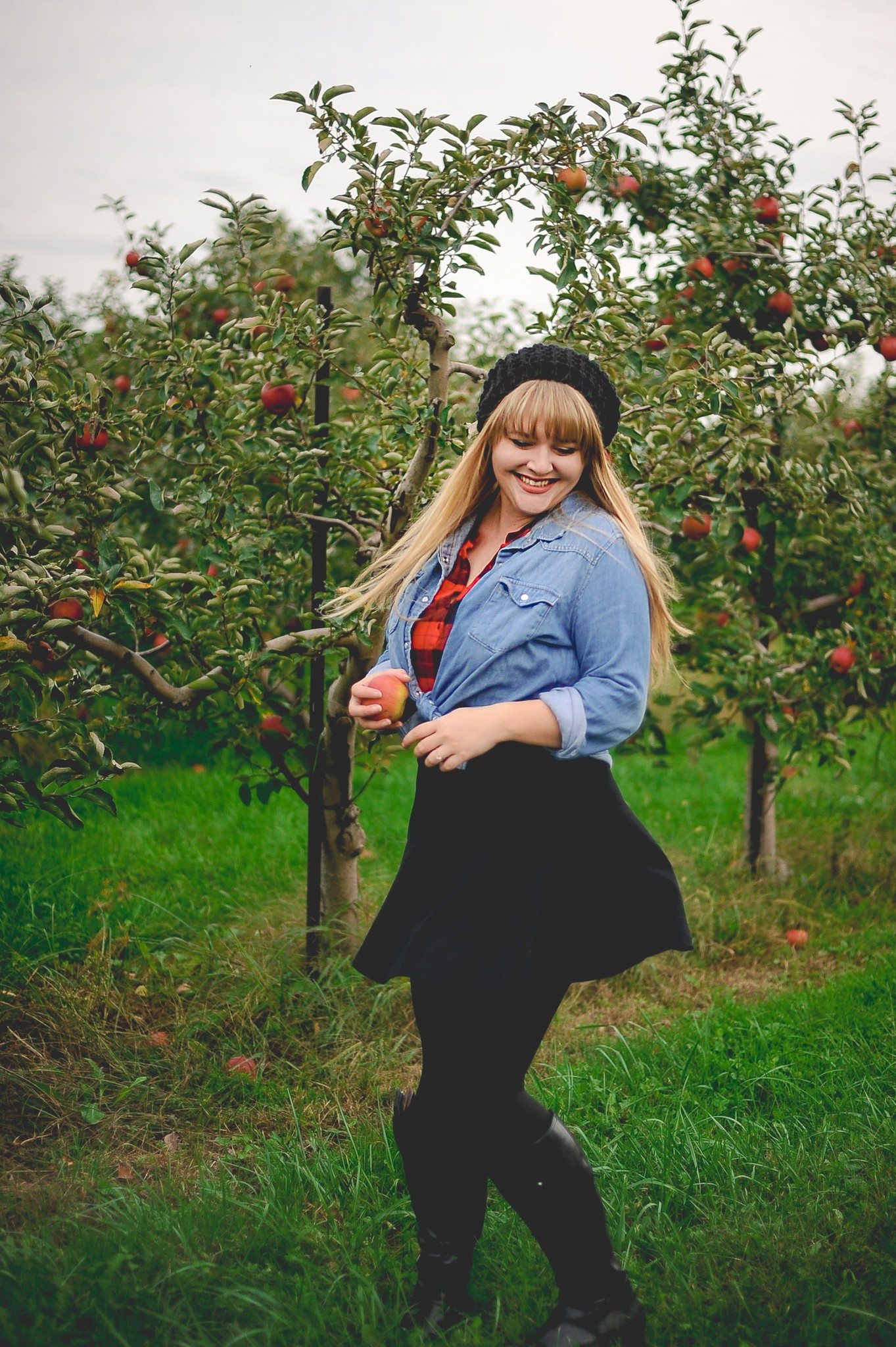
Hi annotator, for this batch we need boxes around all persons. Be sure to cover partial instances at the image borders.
[347,344,700,1347]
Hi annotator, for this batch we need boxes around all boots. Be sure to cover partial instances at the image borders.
[393,1087,489,1345]
[491,1109,647,1347]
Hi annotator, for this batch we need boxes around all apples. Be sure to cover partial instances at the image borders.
[22,200,432,754]
[145,1032,170,1045]
[785,928,808,948]
[223,1055,256,1078]
[558,166,896,720]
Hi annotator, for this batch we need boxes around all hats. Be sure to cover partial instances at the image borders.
[476,343,621,448]
